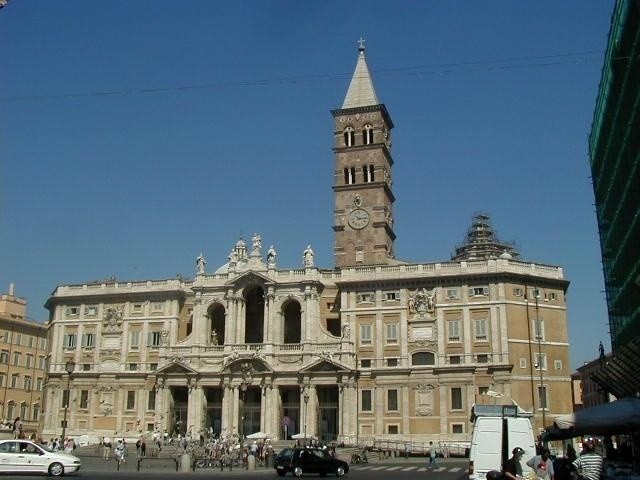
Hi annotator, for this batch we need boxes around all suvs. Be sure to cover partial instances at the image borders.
[273,448,349,478]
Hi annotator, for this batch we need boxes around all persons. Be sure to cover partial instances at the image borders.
[252,231,262,249]
[404,444,410,458]
[197,252,207,273]
[503,447,525,479]
[267,244,277,259]
[442,444,449,461]
[99,419,336,468]
[304,245,313,265]
[527,439,632,480]
[12,416,76,453]
[228,248,238,266]
[424,441,440,468]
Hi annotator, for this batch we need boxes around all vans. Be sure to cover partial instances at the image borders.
[468,415,538,480]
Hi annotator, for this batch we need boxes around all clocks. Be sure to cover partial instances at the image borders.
[348,208,370,230]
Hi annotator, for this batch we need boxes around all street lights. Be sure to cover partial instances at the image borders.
[303,392,310,445]
[237,379,249,468]
[59,357,77,450]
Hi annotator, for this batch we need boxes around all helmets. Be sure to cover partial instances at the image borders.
[512,447,525,455]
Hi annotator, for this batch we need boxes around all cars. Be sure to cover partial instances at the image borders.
[0,439,82,477]
